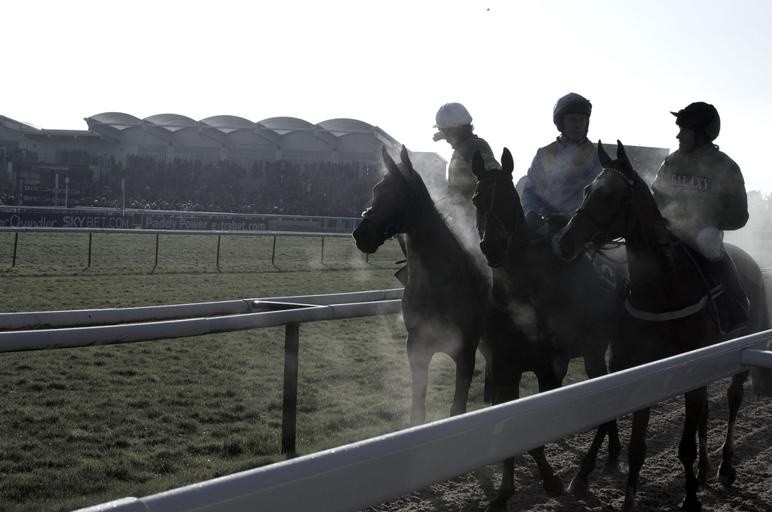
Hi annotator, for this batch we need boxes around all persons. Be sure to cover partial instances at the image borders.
[432,102,501,281]
[1,148,383,228]
[521,91,615,306]
[652,102,750,336]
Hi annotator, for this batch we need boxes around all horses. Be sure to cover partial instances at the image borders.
[353,144,497,427]
[471,146,633,512]
[558,137,769,512]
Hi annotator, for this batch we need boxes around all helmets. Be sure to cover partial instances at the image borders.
[670,102,721,140]
[436,103,473,130]
[553,93,592,131]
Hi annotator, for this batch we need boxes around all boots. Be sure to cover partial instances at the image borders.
[715,251,751,325]
